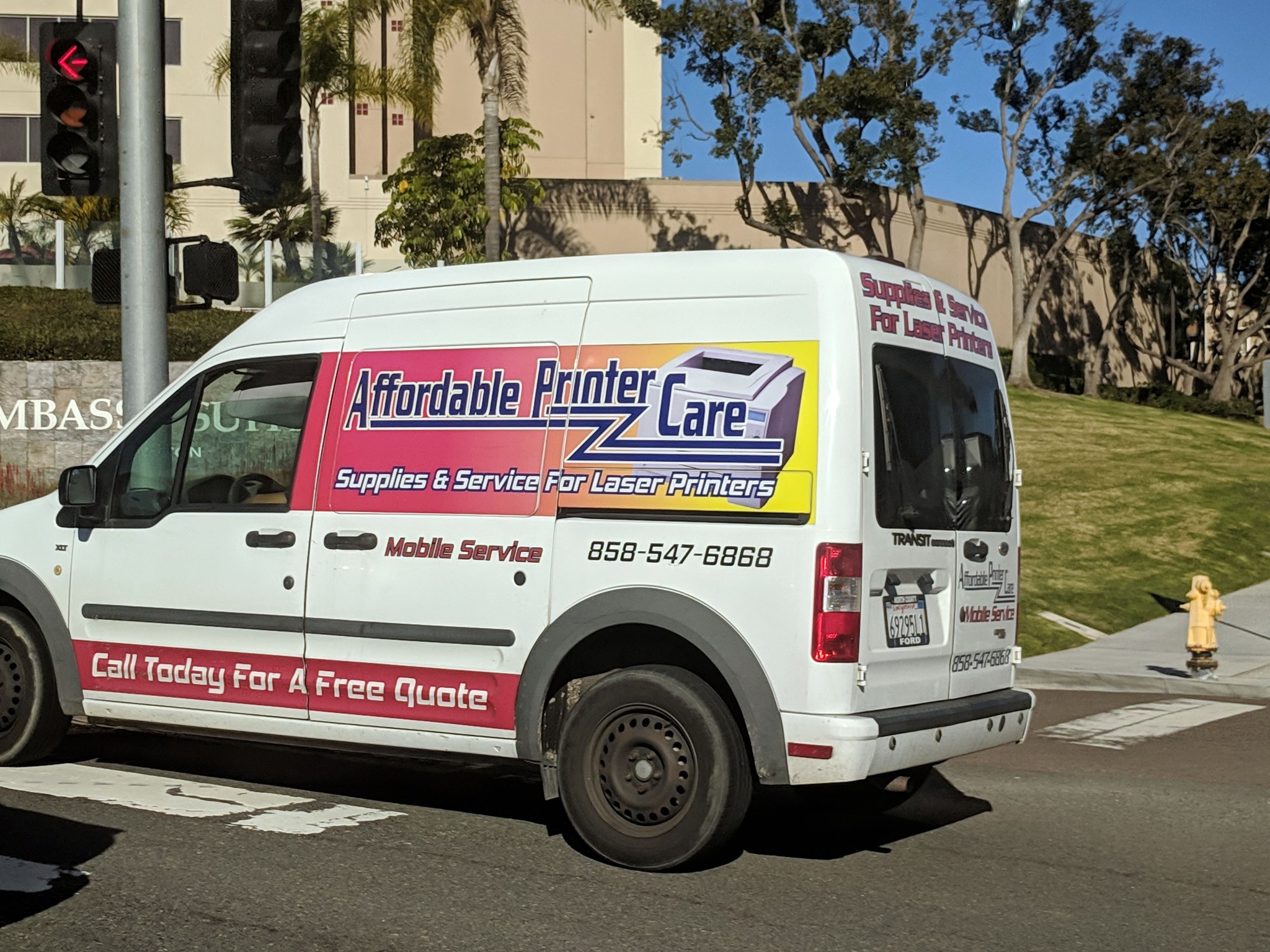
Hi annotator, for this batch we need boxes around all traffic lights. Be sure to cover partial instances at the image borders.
[182,243,242,304]
[231,0,304,196]
[91,247,122,306]
[38,21,120,197]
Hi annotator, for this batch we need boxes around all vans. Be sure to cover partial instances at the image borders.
[0,245,1040,872]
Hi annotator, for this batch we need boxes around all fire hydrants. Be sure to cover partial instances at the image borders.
[1179,573,1227,679]
